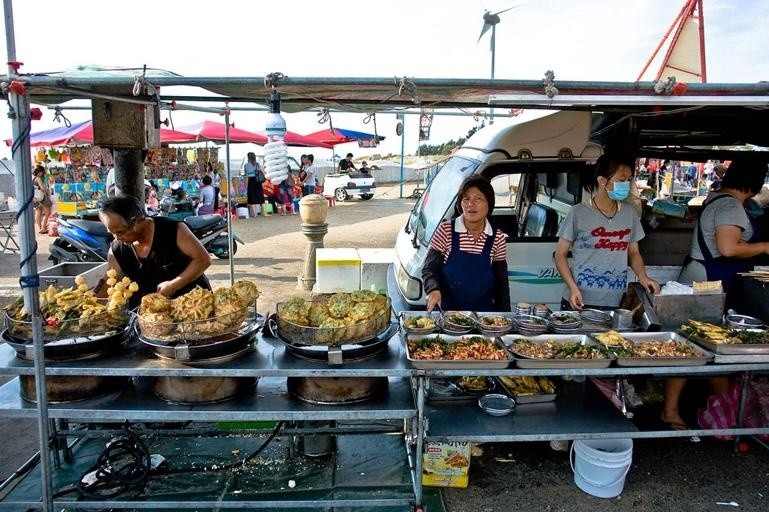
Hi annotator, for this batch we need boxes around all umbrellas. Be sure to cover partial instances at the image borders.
[175,120,269,147]
[1,105,203,146]
[303,128,386,174]
[265,130,334,150]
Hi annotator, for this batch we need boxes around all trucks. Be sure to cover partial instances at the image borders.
[385,110,769,326]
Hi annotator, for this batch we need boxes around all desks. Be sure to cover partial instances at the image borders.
[0,326,418,511]
[423,363,769,443]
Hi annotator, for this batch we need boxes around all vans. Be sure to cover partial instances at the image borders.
[239,153,318,186]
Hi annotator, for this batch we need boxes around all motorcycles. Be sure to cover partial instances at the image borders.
[154,198,245,259]
[48,216,115,266]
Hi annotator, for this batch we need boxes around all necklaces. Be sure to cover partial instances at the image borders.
[586,190,627,226]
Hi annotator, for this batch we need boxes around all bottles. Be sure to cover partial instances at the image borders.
[515,302,531,320]
[533,304,548,317]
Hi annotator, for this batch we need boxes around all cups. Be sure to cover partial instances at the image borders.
[614,310,632,330]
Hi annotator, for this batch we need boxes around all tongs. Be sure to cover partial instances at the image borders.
[429,304,443,319]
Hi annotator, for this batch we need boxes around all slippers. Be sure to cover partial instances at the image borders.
[38,230,50,234]
[665,420,691,433]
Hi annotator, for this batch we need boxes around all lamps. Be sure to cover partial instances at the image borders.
[264,91,289,186]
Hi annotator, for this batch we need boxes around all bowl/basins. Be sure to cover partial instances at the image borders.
[723,314,763,329]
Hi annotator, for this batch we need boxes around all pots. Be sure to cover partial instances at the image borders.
[134,312,265,361]
[269,315,399,358]
[2,312,134,359]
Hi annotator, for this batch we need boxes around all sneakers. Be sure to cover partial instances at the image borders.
[549,436,569,452]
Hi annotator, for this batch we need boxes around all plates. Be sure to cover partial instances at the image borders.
[549,310,639,333]
[501,334,615,369]
[425,377,495,405]
[551,311,581,329]
[440,312,475,328]
[709,352,769,364]
[686,330,769,354]
[437,315,475,335]
[479,394,515,417]
[581,310,613,326]
[606,331,715,367]
[403,323,437,334]
[478,312,551,333]
[513,314,547,333]
[404,334,514,369]
[400,311,483,334]
[480,315,512,333]
[497,377,557,402]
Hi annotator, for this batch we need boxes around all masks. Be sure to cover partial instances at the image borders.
[600,180,631,201]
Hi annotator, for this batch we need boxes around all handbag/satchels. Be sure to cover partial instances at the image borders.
[198,187,216,215]
[256,163,267,185]
[33,189,45,203]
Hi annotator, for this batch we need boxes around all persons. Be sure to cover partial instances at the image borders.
[303,154,315,194]
[659,160,670,190]
[644,158,650,173]
[661,152,769,443]
[300,154,307,176]
[652,160,656,166]
[204,161,214,185]
[704,160,714,175]
[675,163,682,181]
[422,176,545,469]
[699,174,708,196]
[550,153,660,451]
[713,160,732,178]
[684,174,697,201]
[359,161,369,174]
[686,162,697,179]
[337,152,358,200]
[244,152,272,217]
[196,175,215,215]
[666,160,673,172]
[709,176,723,192]
[175,190,195,202]
[30,166,54,234]
[699,163,704,179]
[279,163,298,216]
[647,172,657,201]
[212,169,221,210]
[91,196,214,310]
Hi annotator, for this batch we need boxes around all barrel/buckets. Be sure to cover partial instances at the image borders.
[570,437,633,499]
